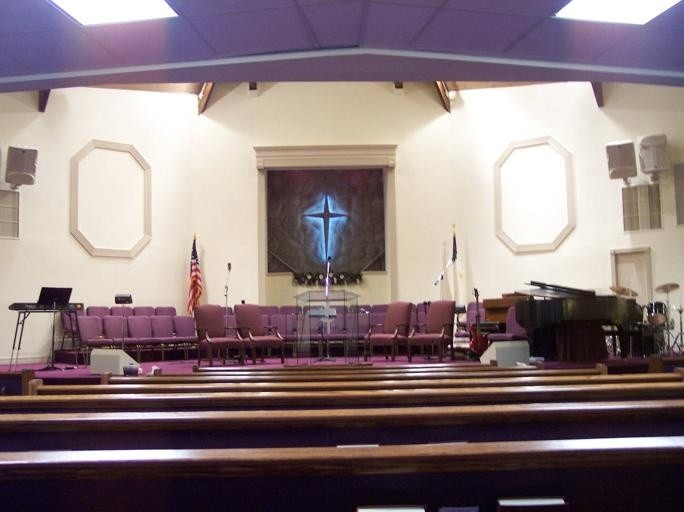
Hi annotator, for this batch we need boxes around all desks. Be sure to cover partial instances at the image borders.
[8,309,61,371]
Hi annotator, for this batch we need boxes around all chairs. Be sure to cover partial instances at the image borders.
[60,301,487,366]
[487,306,528,343]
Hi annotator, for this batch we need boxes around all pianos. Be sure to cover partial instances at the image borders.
[8,302,84,311]
[514,281,638,362]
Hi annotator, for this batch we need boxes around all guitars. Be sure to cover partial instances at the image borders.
[470,289,489,354]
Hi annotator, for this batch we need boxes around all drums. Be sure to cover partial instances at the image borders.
[647,302,667,326]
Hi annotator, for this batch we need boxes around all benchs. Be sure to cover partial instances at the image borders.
[0,368,684,512]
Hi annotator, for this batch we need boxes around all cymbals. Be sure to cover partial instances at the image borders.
[656,283,679,293]
[610,286,637,296]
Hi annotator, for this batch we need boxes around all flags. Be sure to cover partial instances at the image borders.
[187,239,203,318]
[451,235,460,300]
